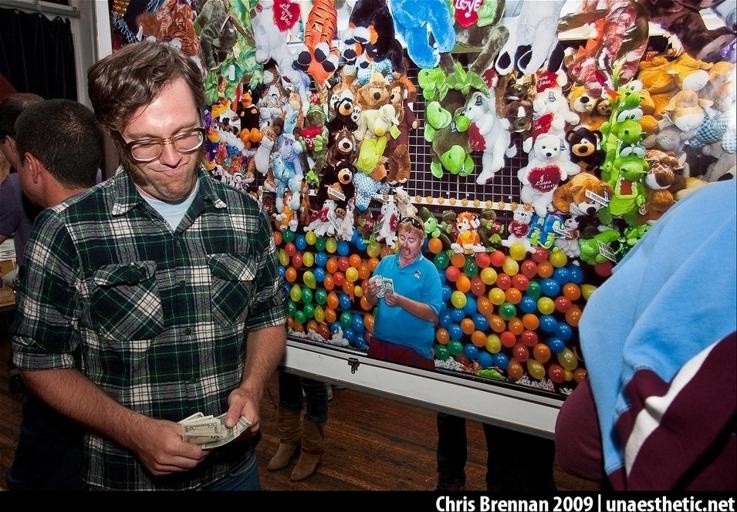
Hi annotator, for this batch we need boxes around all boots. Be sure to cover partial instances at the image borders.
[266,403,324,481]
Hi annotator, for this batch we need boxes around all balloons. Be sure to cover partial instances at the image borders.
[272,217,611,384]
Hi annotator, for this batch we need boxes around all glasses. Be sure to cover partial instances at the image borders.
[400,217,424,233]
[111,126,206,163]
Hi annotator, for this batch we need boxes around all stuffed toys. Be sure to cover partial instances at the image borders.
[110,1,737,265]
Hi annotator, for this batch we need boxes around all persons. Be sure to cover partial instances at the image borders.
[13,40,288,490]
[434,179,737,491]
[302,381,344,401]
[267,370,328,482]
[0,91,102,401]
[6,99,99,493]
